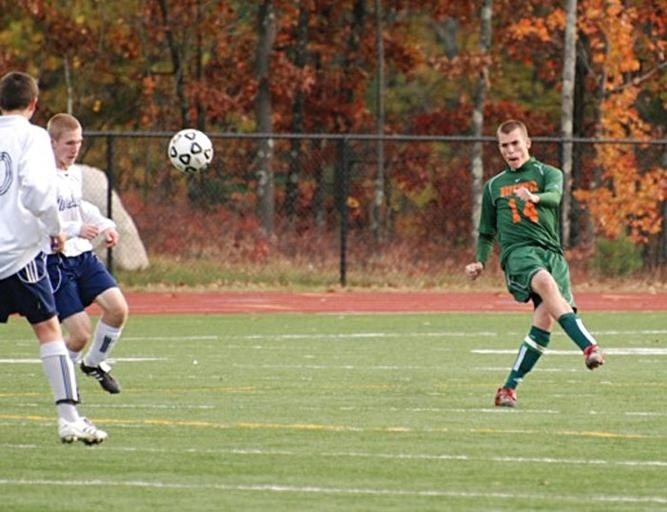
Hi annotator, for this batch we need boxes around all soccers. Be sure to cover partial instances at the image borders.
[166,128,214,175]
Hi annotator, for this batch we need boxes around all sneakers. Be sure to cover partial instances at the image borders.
[80,360,120,393]
[495,388,517,407]
[58,416,107,446]
[583,344,605,369]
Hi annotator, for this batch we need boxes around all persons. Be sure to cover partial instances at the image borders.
[43,112,130,395]
[463,119,605,409]
[0,71,108,447]
